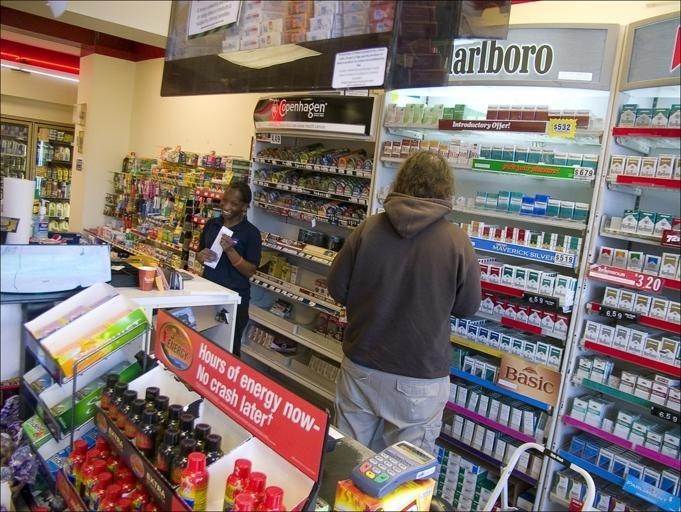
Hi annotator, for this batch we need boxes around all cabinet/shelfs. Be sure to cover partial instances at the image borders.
[32,119,76,235]
[0,114,31,216]
[240,93,380,419]
[542,14,681,510]
[364,21,621,511]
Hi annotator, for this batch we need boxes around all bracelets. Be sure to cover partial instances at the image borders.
[231,256,244,268]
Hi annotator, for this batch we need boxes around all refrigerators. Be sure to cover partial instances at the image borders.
[0,111,74,235]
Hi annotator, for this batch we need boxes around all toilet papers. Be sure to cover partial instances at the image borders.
[3,178,37,246]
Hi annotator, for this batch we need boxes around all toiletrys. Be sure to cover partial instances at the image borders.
[32,198,51,239]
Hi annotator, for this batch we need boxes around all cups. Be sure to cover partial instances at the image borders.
[138,266,157,291]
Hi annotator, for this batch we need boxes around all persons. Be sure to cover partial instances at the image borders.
[195,181,262,356]
[327,148,483,456]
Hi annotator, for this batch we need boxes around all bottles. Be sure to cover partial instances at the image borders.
[37,373,285,512]
